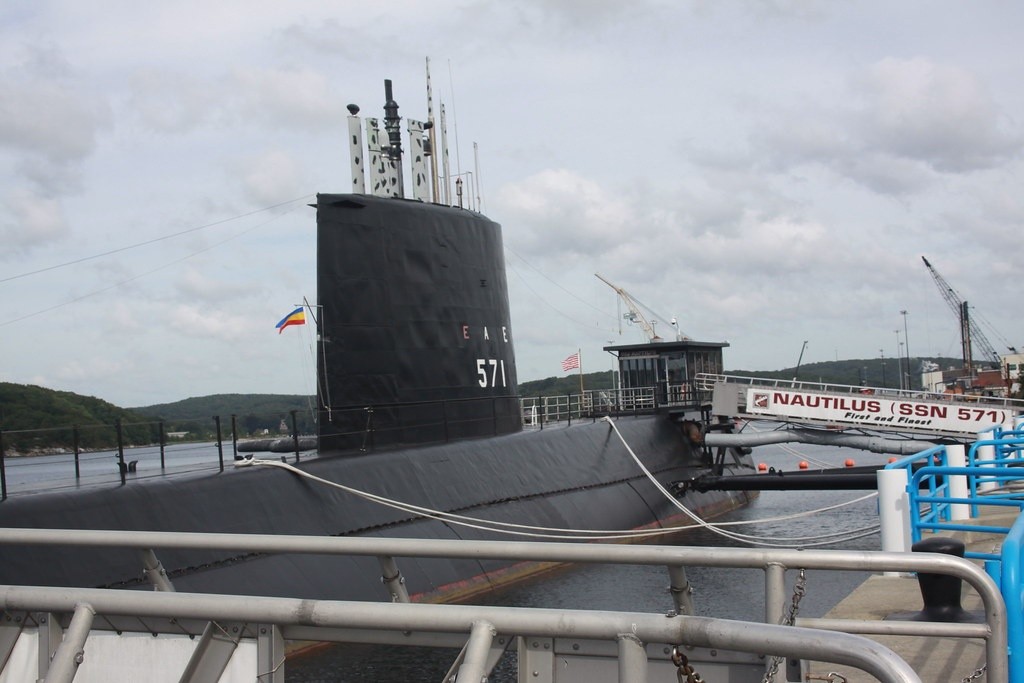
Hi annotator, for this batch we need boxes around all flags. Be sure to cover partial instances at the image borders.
[560,351,581,372]
[275,307,304,334]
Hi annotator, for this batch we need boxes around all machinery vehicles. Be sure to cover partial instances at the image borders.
[595,272,693,342]
[921,255,1019,404]
